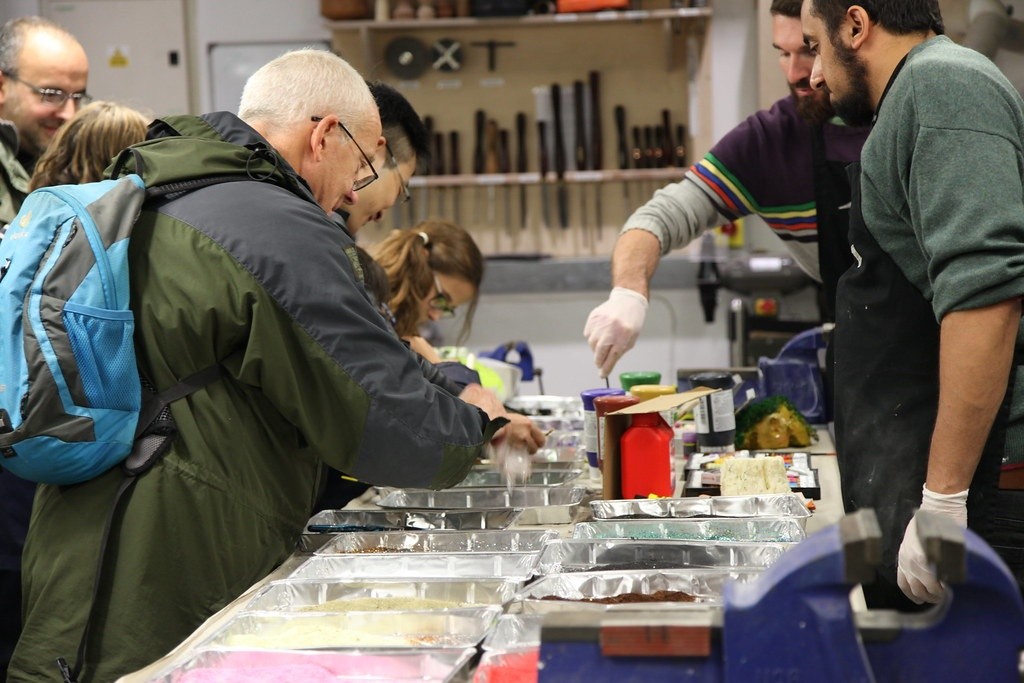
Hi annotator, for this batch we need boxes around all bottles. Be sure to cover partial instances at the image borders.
[578,367,738,503]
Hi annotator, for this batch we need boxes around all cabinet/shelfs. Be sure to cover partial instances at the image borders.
[317,0,723,267]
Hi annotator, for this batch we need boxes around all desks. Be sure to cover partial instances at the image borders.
[110,424,883,683]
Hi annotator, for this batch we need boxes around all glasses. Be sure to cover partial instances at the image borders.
[427,277,456,322]
[312,117,378,192]
[384,142,411,204]
[3,71,93,106]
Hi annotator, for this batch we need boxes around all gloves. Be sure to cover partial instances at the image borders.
[581,285,647,381]
[896,487,971,607]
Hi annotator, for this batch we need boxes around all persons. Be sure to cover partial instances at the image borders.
[0,15,87,239]
[582,1,875,429]
[0,102,155,682]
[800,0,1024,615]
[308,80,545,521]
[372,219,486,362]
[9,48,513,683]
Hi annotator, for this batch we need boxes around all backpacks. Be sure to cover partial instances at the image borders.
[1,169,271,489]
